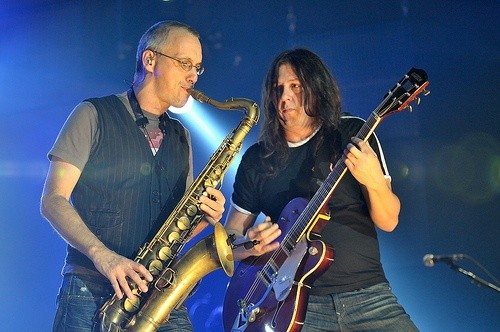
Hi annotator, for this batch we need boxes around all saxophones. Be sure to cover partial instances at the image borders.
[91,87,261,332]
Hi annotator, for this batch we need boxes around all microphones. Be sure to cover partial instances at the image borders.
[227,234,261,249]
[422,253,463,267]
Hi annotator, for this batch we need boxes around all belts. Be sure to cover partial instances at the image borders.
[61,266,114,287]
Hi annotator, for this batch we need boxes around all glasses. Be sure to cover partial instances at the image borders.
[146,48,205,76]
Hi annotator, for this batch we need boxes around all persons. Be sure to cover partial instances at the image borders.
[39,21,226,332]
[224,49,420,332]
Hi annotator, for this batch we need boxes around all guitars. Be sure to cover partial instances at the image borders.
[221,65,433,332]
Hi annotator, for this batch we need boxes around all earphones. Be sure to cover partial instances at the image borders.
[145,58,152,64]
